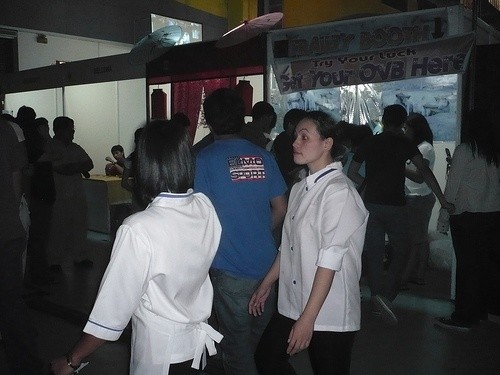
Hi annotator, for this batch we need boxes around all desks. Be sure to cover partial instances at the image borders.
[82,176,133,233]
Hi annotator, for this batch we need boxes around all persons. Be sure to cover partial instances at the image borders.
[399,113,437,290]
[347,104,455,326]
[192,88,288,375]
[121,101,373,279]
[249,111,370,375]
[0,105,93,273]
[105,145,127,177]
[50,120,223,375]
[434,109,500,333]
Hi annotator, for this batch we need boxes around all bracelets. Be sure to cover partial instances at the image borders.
[66,354,81,370]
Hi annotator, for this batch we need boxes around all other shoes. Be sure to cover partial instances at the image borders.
[434,311,472,331]
[51,264,64,278]
[370,294,401,321]
[74,258,94,268]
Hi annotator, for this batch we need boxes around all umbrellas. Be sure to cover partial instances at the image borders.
[129,25,184,65]
[215,12,283,48]
